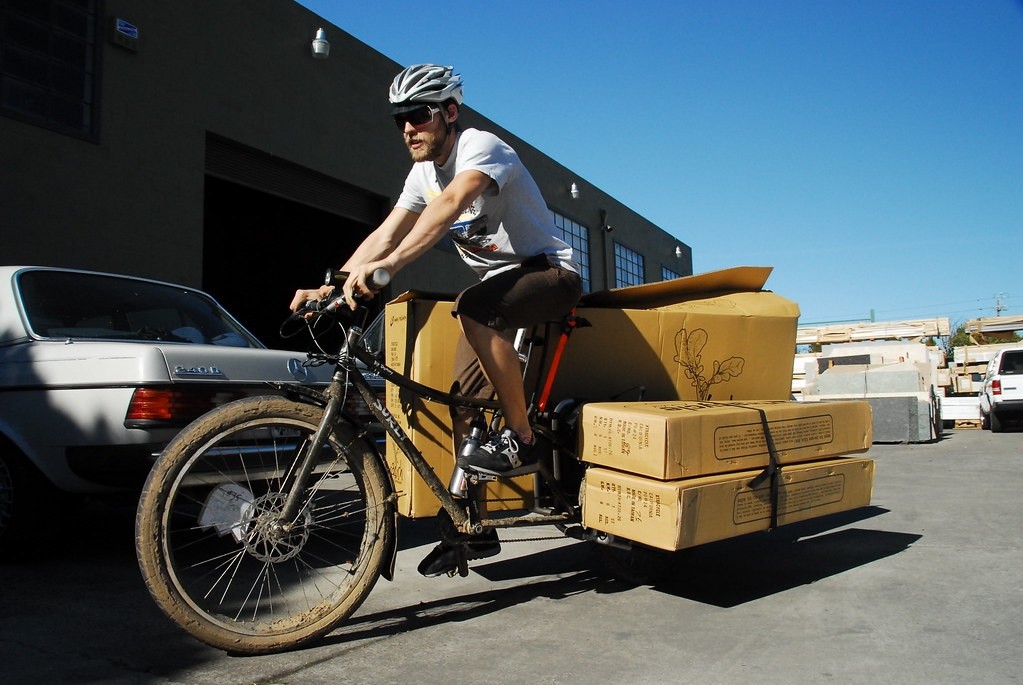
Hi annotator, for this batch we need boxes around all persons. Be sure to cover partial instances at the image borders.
[289,62,584,575]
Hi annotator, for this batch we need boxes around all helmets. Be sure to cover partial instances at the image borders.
[389,63,463,108]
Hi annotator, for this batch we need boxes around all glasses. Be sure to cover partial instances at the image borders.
[392,106,445,133]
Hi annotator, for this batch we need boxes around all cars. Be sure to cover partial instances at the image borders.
[0,263,386,534]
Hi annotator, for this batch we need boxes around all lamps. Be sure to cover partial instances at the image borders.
[676,247,682,258]
[312,27,332,59]
[569,182,579,198]
[606,224,613,232]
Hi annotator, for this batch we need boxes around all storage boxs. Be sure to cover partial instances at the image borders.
[577,457,876,551]
[382,265,799,520]
[579,398,871,482]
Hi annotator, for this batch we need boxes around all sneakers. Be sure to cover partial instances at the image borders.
[417,526,502,577]
[457,425,543,477]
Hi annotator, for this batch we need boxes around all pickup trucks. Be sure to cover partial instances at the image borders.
[977,346,1023,434]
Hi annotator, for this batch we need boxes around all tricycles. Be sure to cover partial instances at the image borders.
[131,266,894,657]
